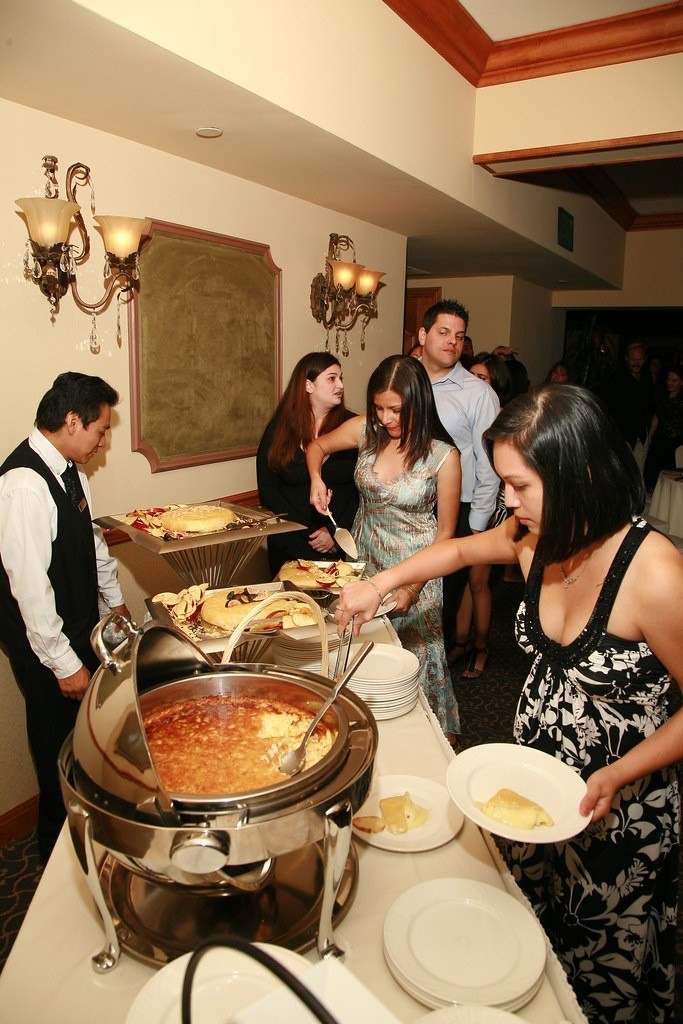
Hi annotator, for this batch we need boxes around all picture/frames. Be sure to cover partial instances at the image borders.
[127,218,284,473]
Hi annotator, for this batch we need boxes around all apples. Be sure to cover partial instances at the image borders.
[125,507,166,536]
[152,583,209,624]
[298,559,353,586]
[265,603,317,629]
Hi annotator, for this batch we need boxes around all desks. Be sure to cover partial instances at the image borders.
[0,580,591,1024]
[648,470,683,539]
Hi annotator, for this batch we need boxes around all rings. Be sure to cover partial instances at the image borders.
[335,605,344,612]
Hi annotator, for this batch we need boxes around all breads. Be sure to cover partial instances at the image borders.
[483,788,554,830]
[279,562,321,588]
[158,505,237,533]
[200,588,291,630]
[380,796,413,835]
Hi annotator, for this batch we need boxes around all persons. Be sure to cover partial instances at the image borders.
[256,352,360,582]
[305,353,463,753]
[408,341,423,360]
[409,296,533,679]
[458,336,576,411]
[333,382,683,1024]
[600,340,683,507]
[1,371,133,867]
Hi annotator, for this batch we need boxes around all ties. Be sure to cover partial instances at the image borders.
[61,464,79,511]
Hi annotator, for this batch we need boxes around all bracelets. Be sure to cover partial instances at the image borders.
[367,579,382,603]
[400,584,421,605]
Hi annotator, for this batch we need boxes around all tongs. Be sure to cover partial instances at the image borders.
[331,616,354,680]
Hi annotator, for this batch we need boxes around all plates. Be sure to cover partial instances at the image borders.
[125,942,312,1024]
[272,626,350,675]
[446,742,594,845]
[328,642,420,721]
[382,876,547,1014]
[417,1005,529,1024]
[352,774,465,853]
[272,559,367,595]
[373,592,398,618]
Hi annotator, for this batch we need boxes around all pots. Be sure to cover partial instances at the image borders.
[56,612,380,974]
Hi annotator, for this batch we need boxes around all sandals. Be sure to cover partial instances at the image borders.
[446,642,467,665]
[463,646,491,679]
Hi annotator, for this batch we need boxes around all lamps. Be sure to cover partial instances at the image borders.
[308,234,383,355]
[18,156,147,355]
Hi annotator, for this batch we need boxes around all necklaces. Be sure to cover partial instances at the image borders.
[559,546,587,588]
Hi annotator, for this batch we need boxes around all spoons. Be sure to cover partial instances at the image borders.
[278,640,374,778]
